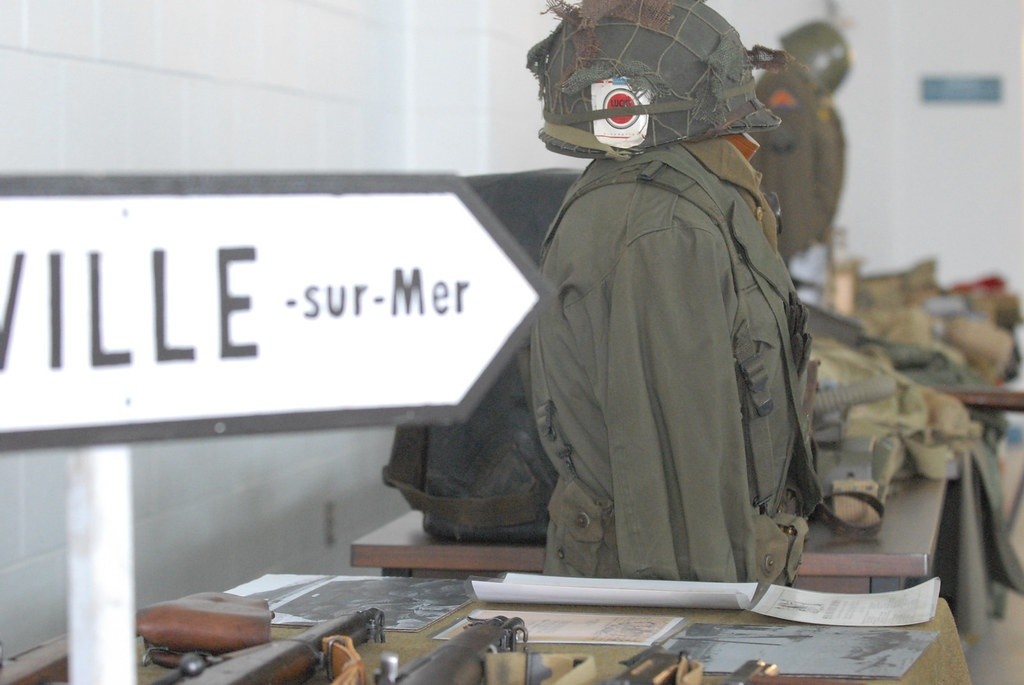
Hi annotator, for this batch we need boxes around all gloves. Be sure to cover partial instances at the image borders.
[781,288,813,377]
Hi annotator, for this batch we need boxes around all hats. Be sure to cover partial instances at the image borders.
[526,0,783,162]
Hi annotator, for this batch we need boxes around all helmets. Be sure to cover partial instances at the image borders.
[780,21,851,93]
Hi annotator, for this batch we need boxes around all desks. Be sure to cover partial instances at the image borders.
[0,385,1024,685]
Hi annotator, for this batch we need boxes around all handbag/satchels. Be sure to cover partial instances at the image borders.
[381,417,558,540]
